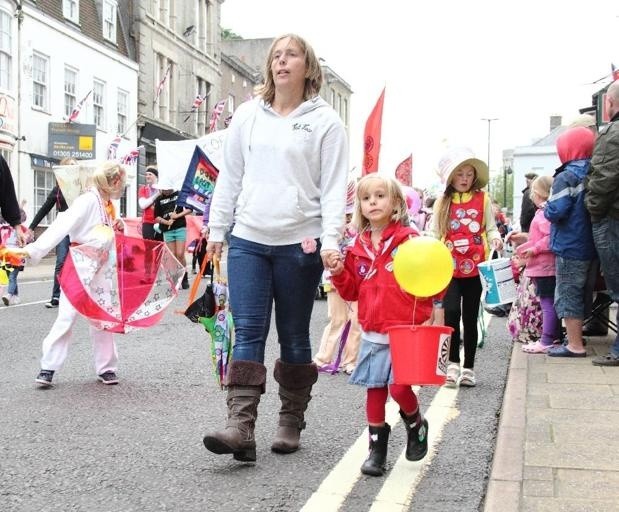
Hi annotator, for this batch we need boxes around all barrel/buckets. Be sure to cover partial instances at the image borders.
[385,297,455,387]
[477,247,518,309]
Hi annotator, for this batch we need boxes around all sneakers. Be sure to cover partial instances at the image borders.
[445,364,460,384]
[591,352,619,366]
[2,296,9,306]
[44,299,59,308]
[96,370,119,384]
[36,370,54,385]
[192,269,197,274]
[460,366,475,386]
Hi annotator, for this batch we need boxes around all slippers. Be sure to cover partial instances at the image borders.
[521,338,586,357]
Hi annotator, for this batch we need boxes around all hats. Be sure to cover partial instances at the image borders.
[433,146,489,195]
[525,174,536,179]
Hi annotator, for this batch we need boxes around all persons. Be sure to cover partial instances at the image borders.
[194,34,344,466]
[326,171,439,481]
[0,123,619,391]
[585,83,619,368]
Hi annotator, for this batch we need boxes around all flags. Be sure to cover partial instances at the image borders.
[360,87,387,176]
[60,62,253,167]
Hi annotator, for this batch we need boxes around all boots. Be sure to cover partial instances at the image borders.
[182,272,189,289]
[361,423,390,476]
[399,405,428,461]
[203,360,267,462]
[271,358,318,453]
